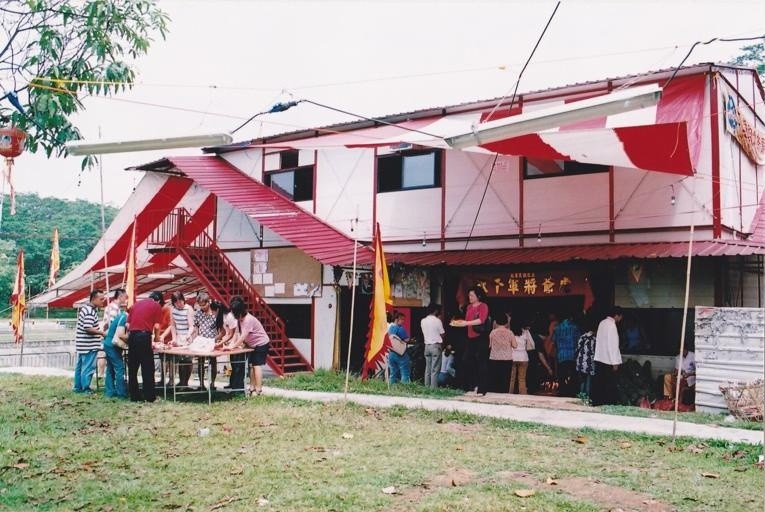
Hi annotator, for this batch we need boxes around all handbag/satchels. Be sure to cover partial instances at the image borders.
[386,335,407,356]
[473,312,492,333]
[111,326,129,349]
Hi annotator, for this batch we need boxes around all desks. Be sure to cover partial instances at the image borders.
[95,339,255,403]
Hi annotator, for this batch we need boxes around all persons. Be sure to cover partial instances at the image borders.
[589,306,624,405]
[487,307,602,400]
[384,304,465,390]
[449,288,492,388]
[72,288,272,402]
[662,341,696,402]
[620,315,648,354]
